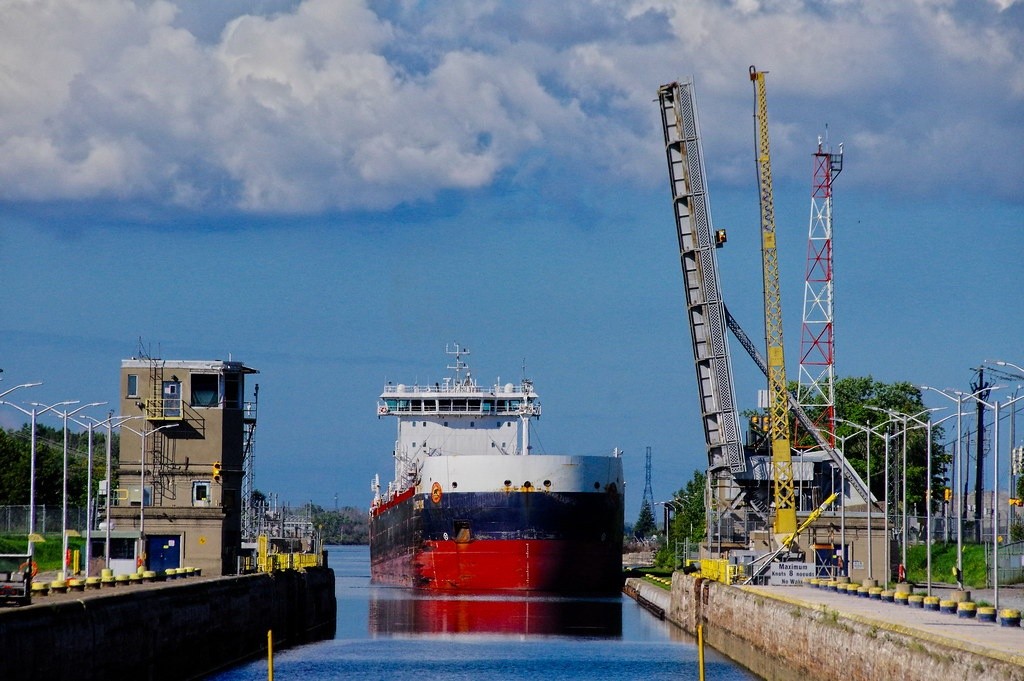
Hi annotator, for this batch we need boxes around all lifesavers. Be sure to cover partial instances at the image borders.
[66,548,72,567]
[19,559,38,578]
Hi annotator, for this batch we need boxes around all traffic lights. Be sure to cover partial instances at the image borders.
[213,462,221,482]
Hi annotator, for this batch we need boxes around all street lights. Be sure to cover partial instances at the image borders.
[58,415,131,578]
[0,401,80,579]
[117,424,179,565]
[79,416,143,568]
[23,402,109,580]
[955,391,1024,610]
[808,406,969,597]
[921,385,999,591]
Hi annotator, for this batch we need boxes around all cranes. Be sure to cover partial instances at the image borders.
[747,65,795,534]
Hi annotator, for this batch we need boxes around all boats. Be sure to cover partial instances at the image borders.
[368,341,623,598]
